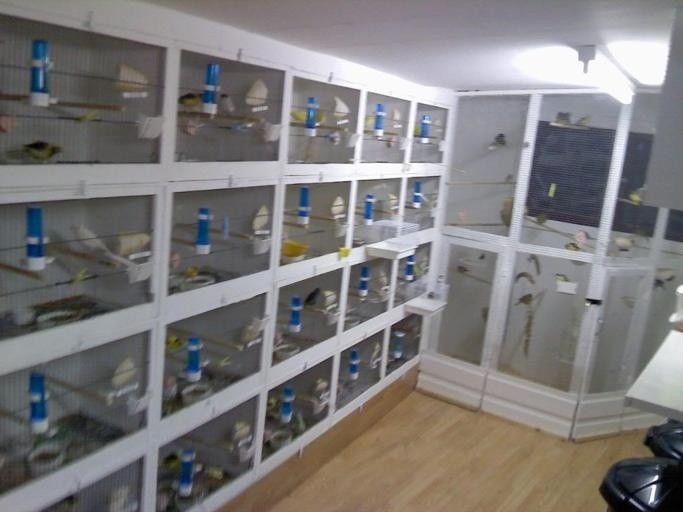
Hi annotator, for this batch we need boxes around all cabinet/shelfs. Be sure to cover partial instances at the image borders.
[420,90,683,438]
[2,5,456,512]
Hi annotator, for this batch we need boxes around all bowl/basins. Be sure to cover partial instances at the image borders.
[27,445,63,478]
[181,384,211,406]
[36,310,81,327]
[274,343,301,360]
[270,431,291,447]
[183,275,215,289]
